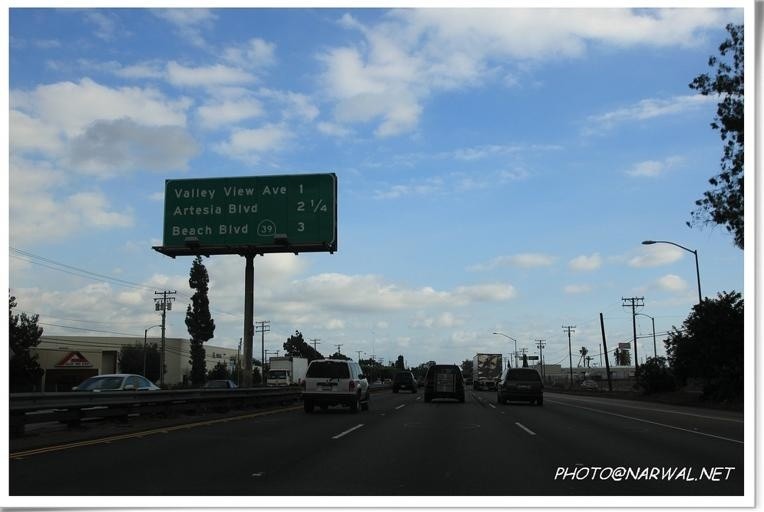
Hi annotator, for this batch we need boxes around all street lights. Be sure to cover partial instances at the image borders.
[635,314,656,358]
[144,325,162,376]
[493,333,518,368]
[642,241,701,303]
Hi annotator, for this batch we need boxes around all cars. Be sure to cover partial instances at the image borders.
[581,380,598,389]
[393,354,544,405]
[72,374,160,392]
[299,360,369,413]
[203,380,238,388]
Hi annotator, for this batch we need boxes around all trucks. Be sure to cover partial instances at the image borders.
[267,357,308,386]
